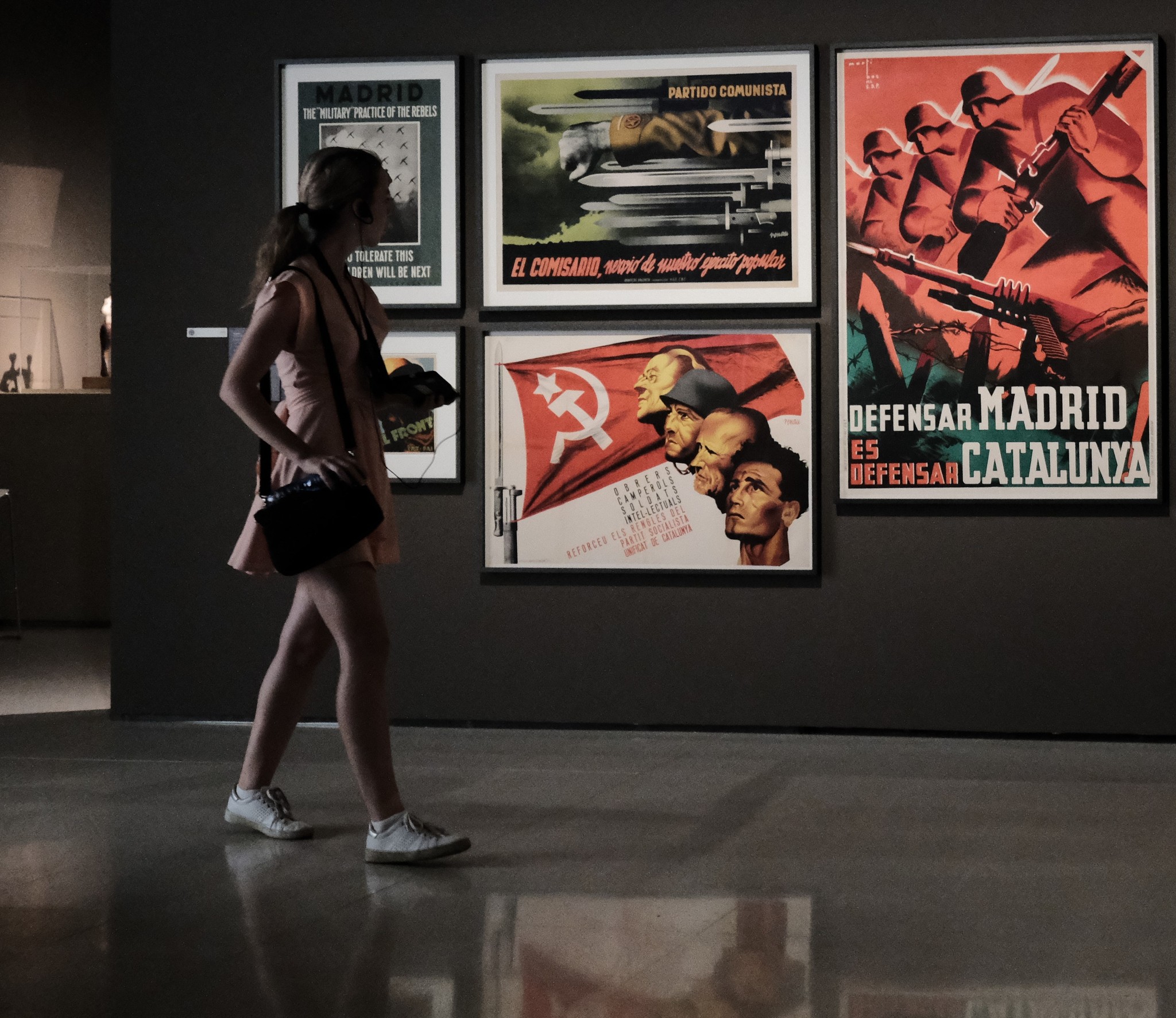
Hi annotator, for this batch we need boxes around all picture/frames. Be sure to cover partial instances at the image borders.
[467,876,837,1018]
[827,28,1173,517]
[253,326,465,492]
[272,52,467,316]
[476,41,819,312]
[472,317,822,579]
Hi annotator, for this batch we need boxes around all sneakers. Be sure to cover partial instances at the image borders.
[367,865,471,911]
[224,783,316,839]
[222,831,305,878]
[363,809,473,865]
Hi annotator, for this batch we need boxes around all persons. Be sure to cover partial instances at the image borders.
[213,148,471,863]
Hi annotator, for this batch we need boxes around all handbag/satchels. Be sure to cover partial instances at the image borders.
[254,467,386,578]
